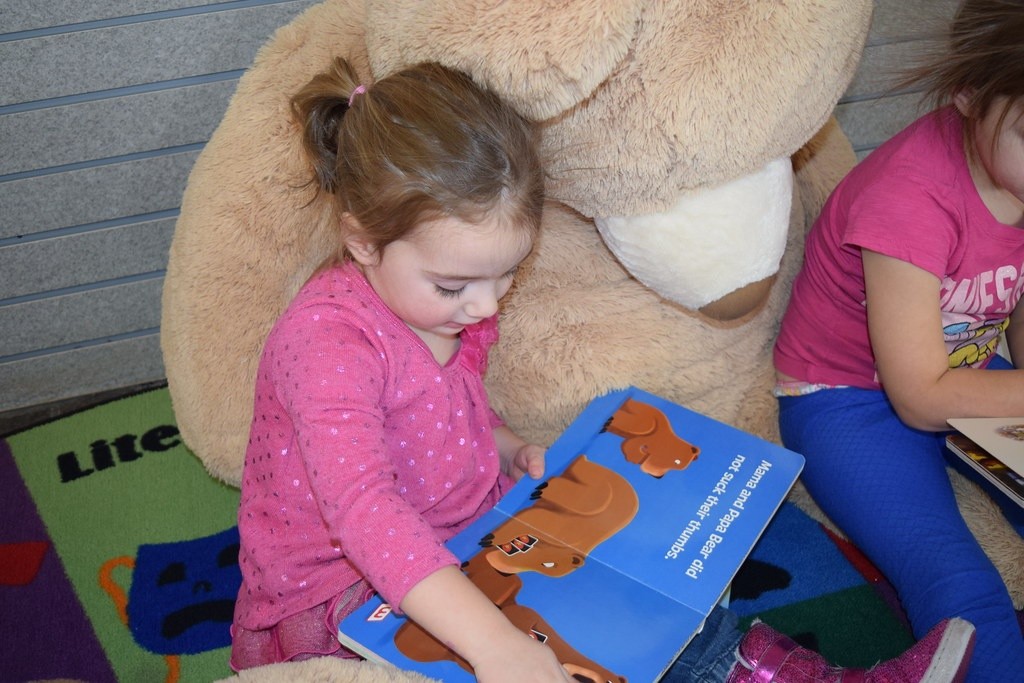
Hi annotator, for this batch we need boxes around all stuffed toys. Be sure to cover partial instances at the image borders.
[161,0,1024,613]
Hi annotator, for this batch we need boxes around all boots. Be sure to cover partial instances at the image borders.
[721,617,976,683]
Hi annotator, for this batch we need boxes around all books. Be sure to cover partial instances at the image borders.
[338,385,805,683]
[946,418,1024,506]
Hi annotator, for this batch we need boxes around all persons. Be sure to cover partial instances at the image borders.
[230,56,976,683]
[774,0,1024,683]
